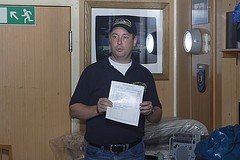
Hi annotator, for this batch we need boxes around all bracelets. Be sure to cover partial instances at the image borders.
[97,107,99,114]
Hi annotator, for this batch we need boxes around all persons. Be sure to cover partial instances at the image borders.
[69,17,162,160]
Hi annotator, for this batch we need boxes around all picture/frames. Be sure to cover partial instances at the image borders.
[90,8,164,74]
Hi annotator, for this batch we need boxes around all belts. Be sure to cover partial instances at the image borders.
[89,139,143,154]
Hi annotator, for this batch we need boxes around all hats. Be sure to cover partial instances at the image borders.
[109,17,137,36]
